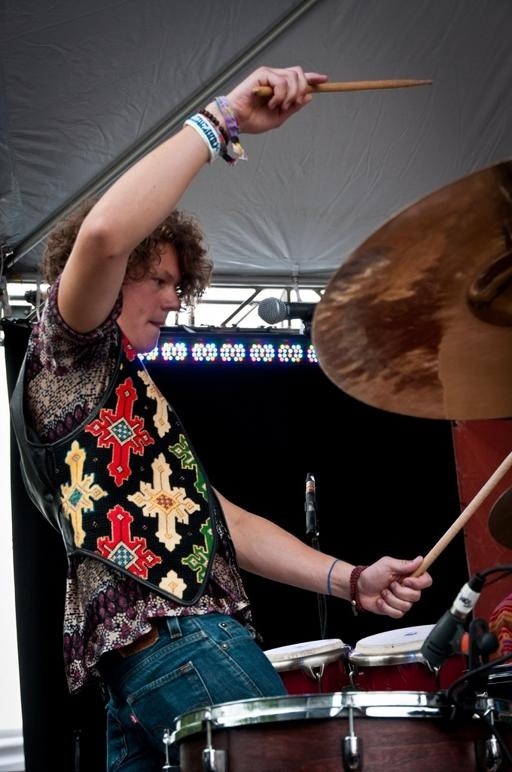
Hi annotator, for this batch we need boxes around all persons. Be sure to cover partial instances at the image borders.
[10,63,434,772]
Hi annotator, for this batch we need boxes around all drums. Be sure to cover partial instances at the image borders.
[349,624,439,692]
[164,690,498,769]
[263,639,350,693]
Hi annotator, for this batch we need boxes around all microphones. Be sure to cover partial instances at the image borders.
[305,472,317,533]
[257,297,318,324]
[420,571,487,668]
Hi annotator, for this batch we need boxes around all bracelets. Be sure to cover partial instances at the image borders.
[327,559,340,597]
[183,96,249,168]
[350,565,367,616]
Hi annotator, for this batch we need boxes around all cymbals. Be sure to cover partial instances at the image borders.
[312,162,510,420]
